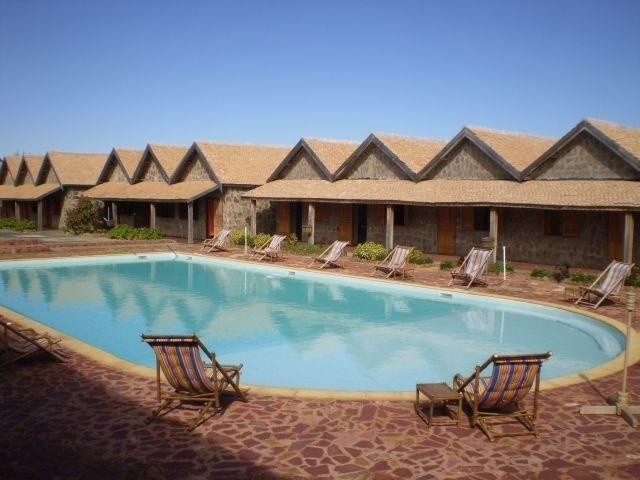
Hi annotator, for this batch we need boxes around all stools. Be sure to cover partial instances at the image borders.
[414,381,466,431]
[563,285,584,303]
[394,266,415,281]
[266,250,286,263]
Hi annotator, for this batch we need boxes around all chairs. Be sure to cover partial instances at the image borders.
[573,259,637,311]
[1,319,71,372]
[451,352,553,443]
[447,246,496,290]
[371,245,416,279]
[139,332,245,434]
[199,229,235,254]
[307,239,351,270]
[247,234,288,263]
